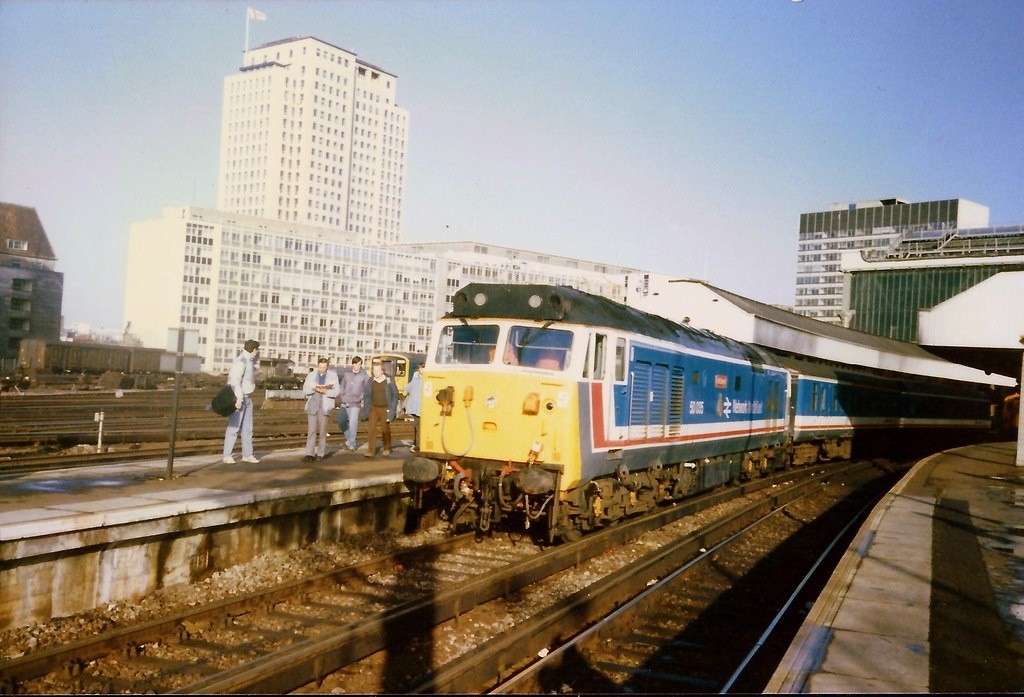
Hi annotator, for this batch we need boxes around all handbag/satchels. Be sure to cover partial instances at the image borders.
[396,400,405,418]
[212,385,237,417]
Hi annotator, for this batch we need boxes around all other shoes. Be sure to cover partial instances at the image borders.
[242,458,259,464]
[365,453,375,457]
[382,450,391,456]
[303,456,316,462]
[223,458,235,464]
[409,445,416,452]
[316,457,324,461]
[345,441,357,451]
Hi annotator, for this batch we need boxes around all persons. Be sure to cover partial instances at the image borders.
[222,339,263,464]
[338,356,369,450]
[302,358,341,462]
[363,361,398,457]
[401,361,425,452]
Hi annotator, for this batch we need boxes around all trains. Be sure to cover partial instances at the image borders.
[369,351,428,401]
[15,339,165,376]
[401,281,1023,543]
